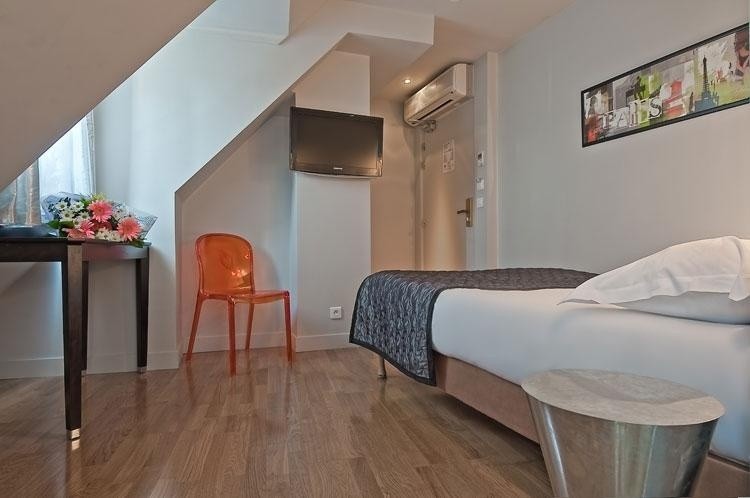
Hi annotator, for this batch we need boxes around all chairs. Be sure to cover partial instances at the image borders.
[185,233,293,377]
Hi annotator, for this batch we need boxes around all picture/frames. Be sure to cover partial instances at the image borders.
[581,24,750,147]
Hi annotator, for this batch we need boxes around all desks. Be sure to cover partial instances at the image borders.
[0,237,152,440]
[521,368,727,498]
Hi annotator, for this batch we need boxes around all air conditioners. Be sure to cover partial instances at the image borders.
[403,62,474,129]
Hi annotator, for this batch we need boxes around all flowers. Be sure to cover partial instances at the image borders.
[40,192,159,249]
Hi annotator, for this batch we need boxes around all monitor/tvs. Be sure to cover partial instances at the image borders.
[288,106,384,178]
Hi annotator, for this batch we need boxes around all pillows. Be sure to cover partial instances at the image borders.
[558,238,750,327]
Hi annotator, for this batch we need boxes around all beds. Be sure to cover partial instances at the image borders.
[348,266,750,498]
